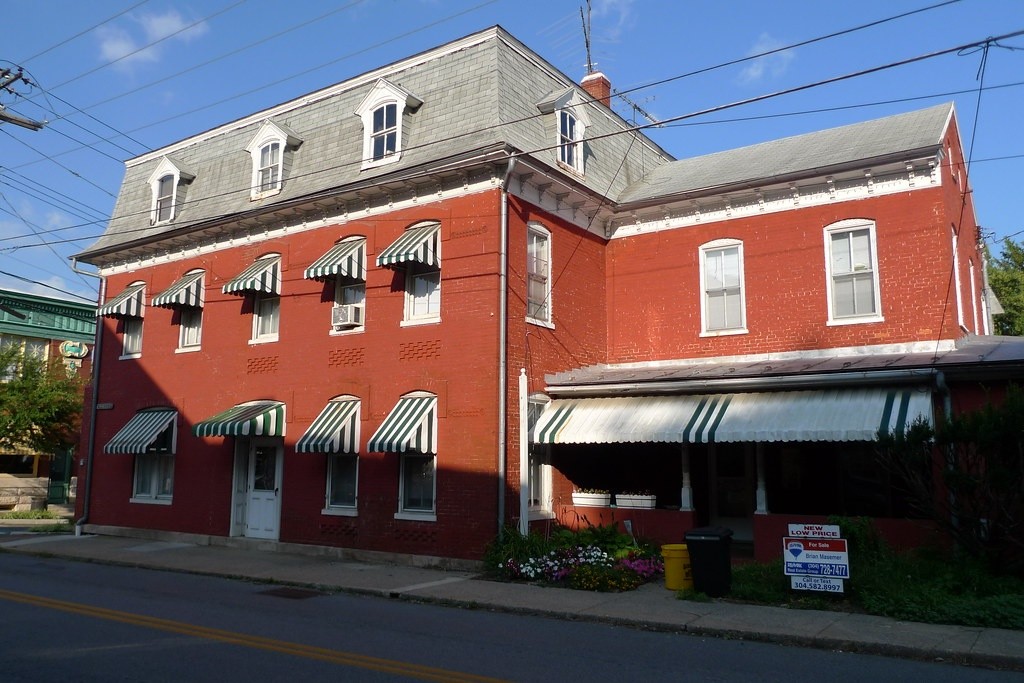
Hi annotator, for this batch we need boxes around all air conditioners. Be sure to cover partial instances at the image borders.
[331,305,364,326]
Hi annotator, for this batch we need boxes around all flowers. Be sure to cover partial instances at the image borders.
[483,509,665,593]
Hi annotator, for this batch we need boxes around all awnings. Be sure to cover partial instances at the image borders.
[103,411,177,455]
[190,404,287,437]
[223,257,281,297]
[295,400,362,453]
[304,239,367,282]
[94,284,146,321]
[151,272,204,309]
[377,223,442,269]
[367,396,438,453]
[527,380,941,446]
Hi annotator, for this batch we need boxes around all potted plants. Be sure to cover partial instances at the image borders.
[615,490,656,508]
[572,488,611,506]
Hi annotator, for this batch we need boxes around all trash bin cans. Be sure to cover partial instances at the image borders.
[681,526,735,599]
